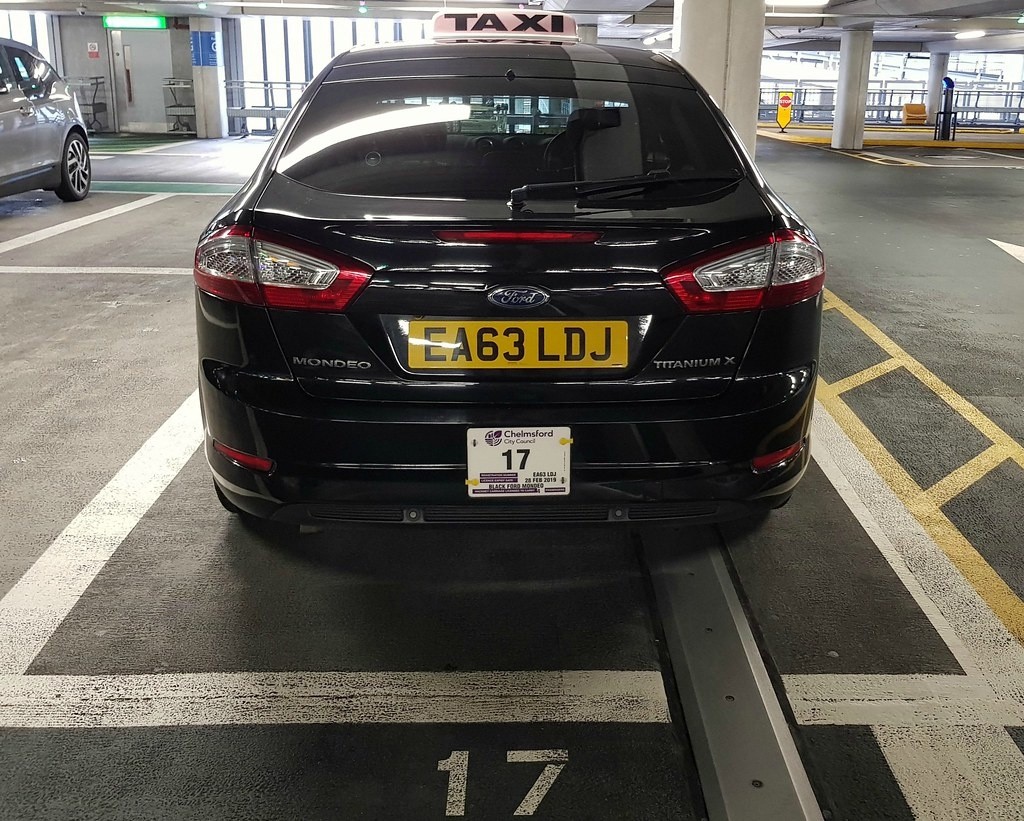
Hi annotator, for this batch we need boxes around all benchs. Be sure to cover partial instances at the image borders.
[903,104,928,125]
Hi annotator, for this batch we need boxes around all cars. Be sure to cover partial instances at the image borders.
[0,37,92,202]
[191,6,828,528]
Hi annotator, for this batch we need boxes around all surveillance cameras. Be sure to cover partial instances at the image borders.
[76,7,86,15]
